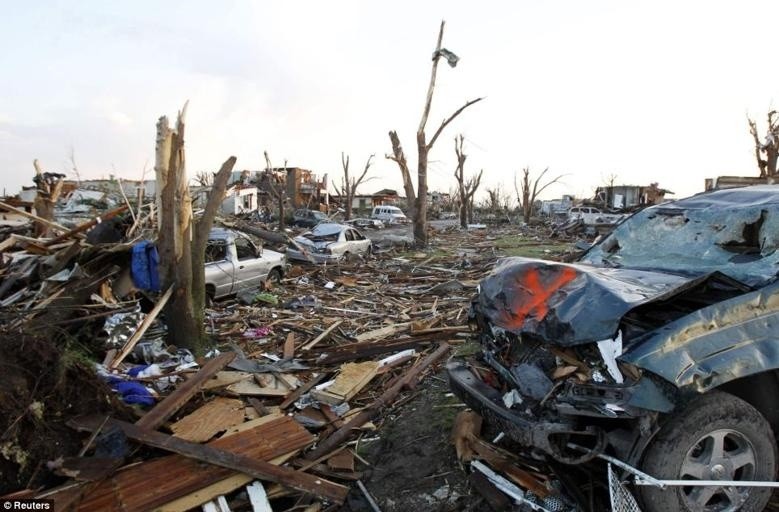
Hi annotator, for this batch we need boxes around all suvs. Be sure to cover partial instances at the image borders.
[442,183,779,512]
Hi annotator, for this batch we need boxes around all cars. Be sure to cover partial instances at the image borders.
[204,226,287,302]
[289,224,373,267]
[286,208,332,227]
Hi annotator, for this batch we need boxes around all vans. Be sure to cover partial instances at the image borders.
[372,204,407,227]
[569,206,604,223]
